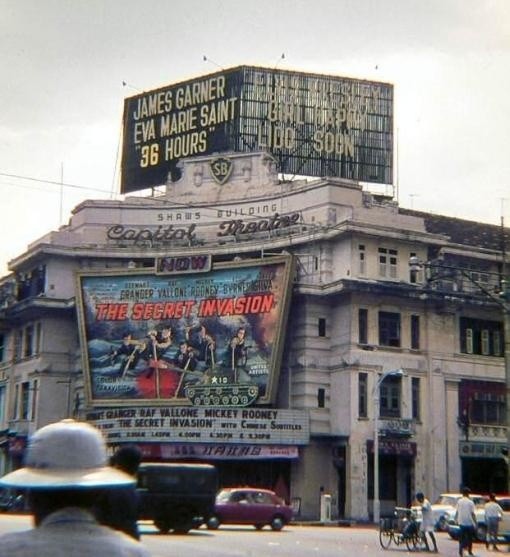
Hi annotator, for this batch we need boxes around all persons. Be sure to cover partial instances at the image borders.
[108,324,248,380]
[0,417,151,557]
[453,486,479,557]
[416,492,438,553]
[90,443,144,541]
[483,492,504,552]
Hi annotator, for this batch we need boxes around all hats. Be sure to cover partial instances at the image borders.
[1,417,139,489]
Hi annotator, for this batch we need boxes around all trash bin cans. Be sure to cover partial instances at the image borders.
[320,494,332,521]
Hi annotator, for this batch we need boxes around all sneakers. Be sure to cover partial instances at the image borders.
[421,539,500,556]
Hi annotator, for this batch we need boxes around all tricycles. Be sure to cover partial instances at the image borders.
[375,505,425,552]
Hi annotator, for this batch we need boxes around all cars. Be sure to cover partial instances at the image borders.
[193,484,296,532]
[409,492,486,531]
[444,495,510,546]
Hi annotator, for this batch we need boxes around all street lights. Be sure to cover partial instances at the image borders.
[371,366,408,524]
[407,257,510,315]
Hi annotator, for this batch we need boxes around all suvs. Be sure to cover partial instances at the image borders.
[134,460,218,535]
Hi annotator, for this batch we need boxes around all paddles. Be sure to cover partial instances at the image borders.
[149,359,204,376]
